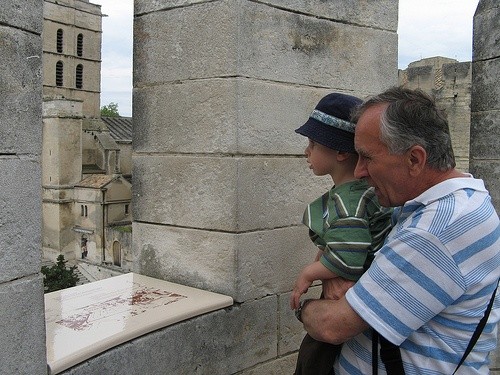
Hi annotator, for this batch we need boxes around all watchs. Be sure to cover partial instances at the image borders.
[294,296,313,322]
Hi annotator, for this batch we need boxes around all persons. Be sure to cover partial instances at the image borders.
[295,96,394,374]
[288,82,500,375]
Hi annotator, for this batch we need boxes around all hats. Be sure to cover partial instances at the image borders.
[295,93,363,156]
